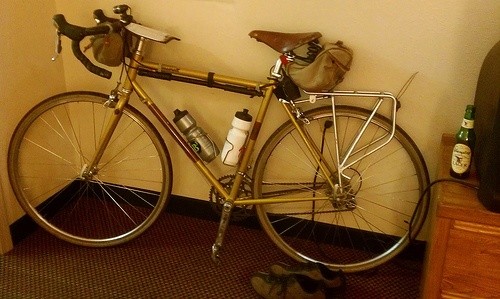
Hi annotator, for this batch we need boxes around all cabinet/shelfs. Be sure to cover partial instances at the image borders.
[421,133,500,299]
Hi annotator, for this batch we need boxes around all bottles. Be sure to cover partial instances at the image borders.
[450,105,475,180]
[221,109,252,166]
[173,109,220,163]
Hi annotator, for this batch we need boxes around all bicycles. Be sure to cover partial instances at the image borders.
[5,5,430,275]
